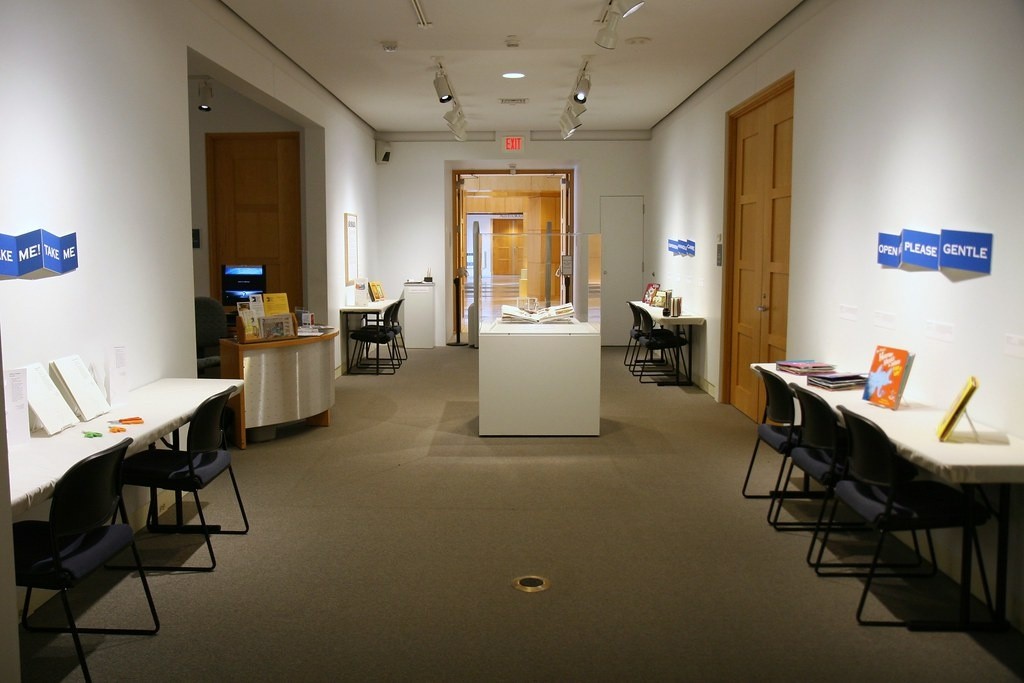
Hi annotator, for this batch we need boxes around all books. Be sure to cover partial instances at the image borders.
[777,359,838,376]
[49,354,111,420]
[237,292,295,340]
[642,283,681,317]
[862,346,916,411]
[502,301,575,324]
[937,377,979,441]
[15,363,81,435]
[807,374,868,392]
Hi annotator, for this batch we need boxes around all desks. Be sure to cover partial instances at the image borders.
[221,321,340,447]
[748,359,1024,630]
[9,378,245,534]
[479,317,600,436]
[341,299,399,374]
[626,300,705,387]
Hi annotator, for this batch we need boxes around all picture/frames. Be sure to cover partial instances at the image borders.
[345,213,358,286]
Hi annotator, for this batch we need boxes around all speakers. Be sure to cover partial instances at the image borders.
[377,141,392,164]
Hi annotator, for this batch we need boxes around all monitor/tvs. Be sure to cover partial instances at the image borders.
[221,265,266,306]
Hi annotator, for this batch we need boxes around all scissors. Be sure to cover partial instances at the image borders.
[82,431,102,438]
[109,427,126,434]
[108,417,144,425]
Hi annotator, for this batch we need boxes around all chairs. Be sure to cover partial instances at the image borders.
[624,302,691,387]
[194,295,234,372]
[10,438,162,683]
[107,386,250,572]
[741,367,1009,637]
[349,299,408,375]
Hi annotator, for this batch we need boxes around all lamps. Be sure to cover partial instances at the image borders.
[594,6,622,49]
[198,79,213,111]
[433,76,453,103]
[443,102,467,141]
[559,74,591,139]
